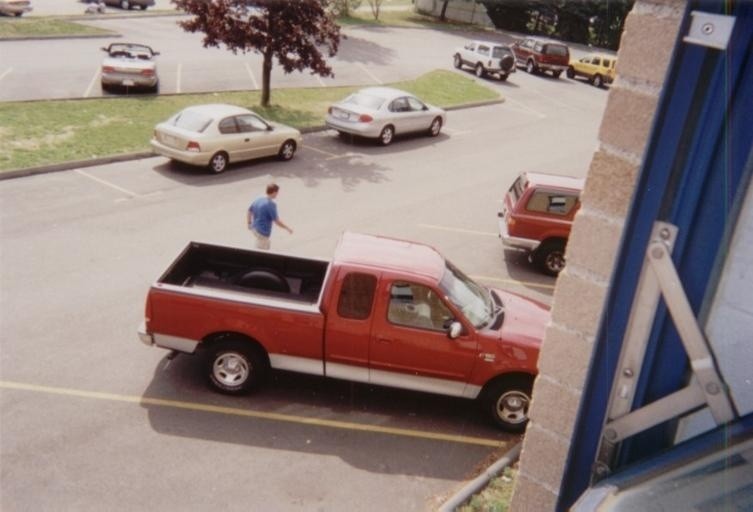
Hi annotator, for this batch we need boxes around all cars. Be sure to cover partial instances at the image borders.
[495,171,586,278]
[150,103,304,174]
[0,0,31,17]
[99,42,161,92]
[566,54,617,87]
[325,86,447,147]
[80,1,154,10]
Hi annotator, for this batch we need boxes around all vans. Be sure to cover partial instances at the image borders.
[509,37,570,78]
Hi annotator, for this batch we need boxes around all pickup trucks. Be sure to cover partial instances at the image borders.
[136,228,551,432]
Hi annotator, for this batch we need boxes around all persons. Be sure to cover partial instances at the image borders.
[84,0,106,13]
[247,185,293,250]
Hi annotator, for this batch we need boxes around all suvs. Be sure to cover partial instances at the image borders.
[453,40,517,80]
